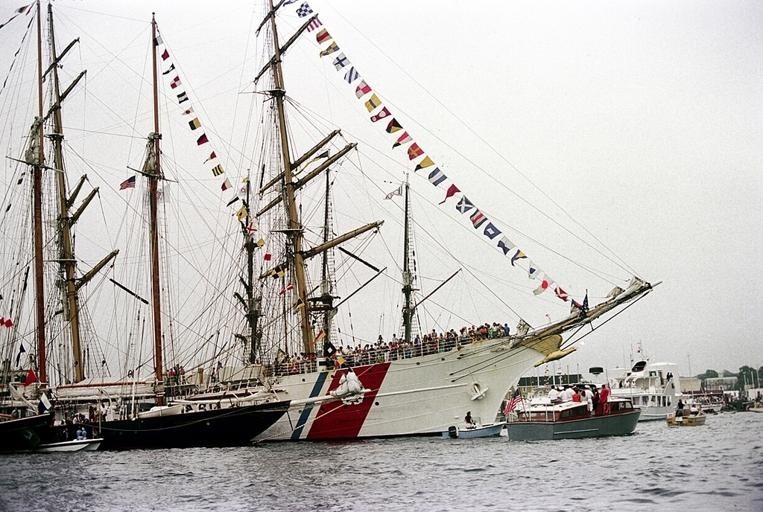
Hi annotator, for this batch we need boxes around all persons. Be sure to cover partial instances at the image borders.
[674,399,700,417]
[549,381,610,418]
[66,404,107,442]
[278,322,509,375]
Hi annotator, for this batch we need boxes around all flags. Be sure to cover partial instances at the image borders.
[0,1,40,97]
[294,1,582,313]
[504,389,521,414]
[0,317,14,328]
[144,2,274,372]
[24,368,37,386]
[17,178,24,185]
[6,204,11,212]
[21,172,25,176]
[120,175,135,190]
